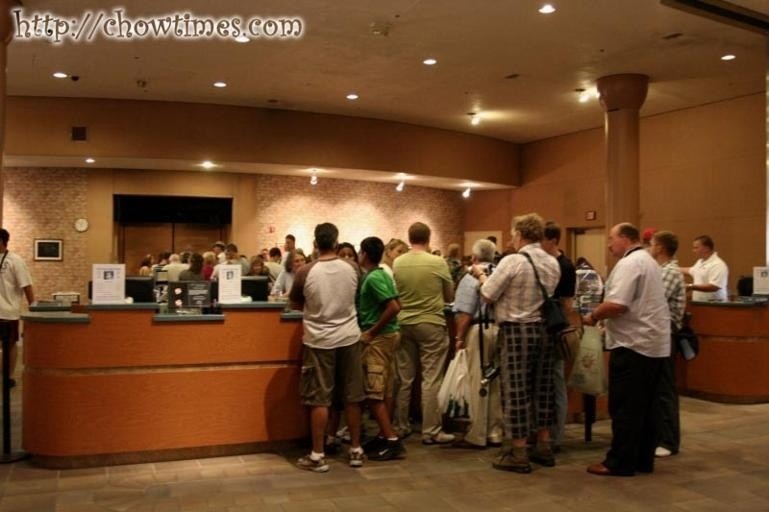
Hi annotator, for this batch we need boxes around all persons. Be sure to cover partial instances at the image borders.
[262,214,728,477]
[140,241,271,283]
[0,228,37,388]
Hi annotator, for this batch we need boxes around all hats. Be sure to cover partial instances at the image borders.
[212,241,225,247]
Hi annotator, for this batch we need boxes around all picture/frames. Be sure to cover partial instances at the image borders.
[34,238,64,261]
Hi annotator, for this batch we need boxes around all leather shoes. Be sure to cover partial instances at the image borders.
[455,435,503,449]
[587,463,611,474]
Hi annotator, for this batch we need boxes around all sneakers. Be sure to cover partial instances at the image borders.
[7,377,15,387]
[296,455,331,472]
[345,450,365,466]
[325,424,368,452]
[423,430,456,445]
[654,446,672,456]
[363,434,408,460]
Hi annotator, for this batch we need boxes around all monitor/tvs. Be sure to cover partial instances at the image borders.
[126,277,154,303]
[241,276,268,302]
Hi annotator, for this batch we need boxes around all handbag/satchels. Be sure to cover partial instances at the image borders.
[541,298,568,331]
[437,349,473,422]
[671,326,699,361]
[558,328,580,365]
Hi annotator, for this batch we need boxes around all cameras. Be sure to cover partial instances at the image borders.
[466,263,495,276]
[481,366,501,387]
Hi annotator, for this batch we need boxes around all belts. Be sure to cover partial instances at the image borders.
[469,319,495,326]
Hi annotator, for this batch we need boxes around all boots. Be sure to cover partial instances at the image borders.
[492,445,531,474]
[529,439,556,466]
